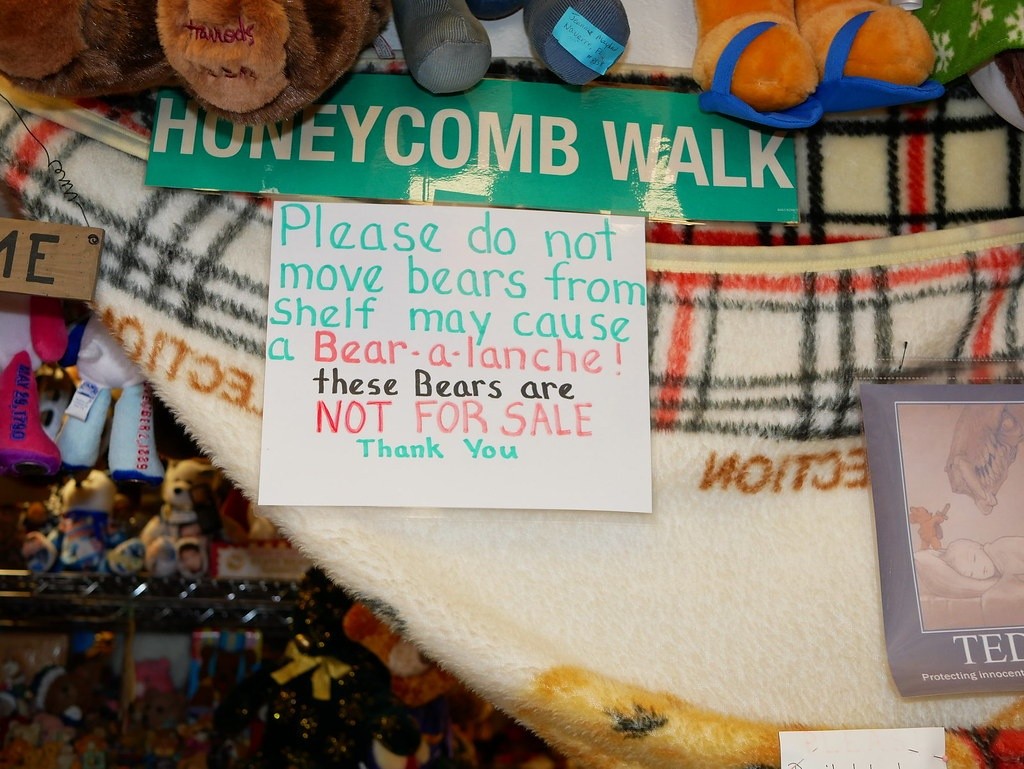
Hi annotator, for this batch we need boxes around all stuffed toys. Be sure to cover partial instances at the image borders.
[385,0,630,98]
[342,601,557,768]
[0,453,373,769]
[1,287,68,480]
[688,1,948,133]
[0,0,386,128]
[54,304,166,488]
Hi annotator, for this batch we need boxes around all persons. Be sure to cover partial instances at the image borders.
[177,539,203,574]
